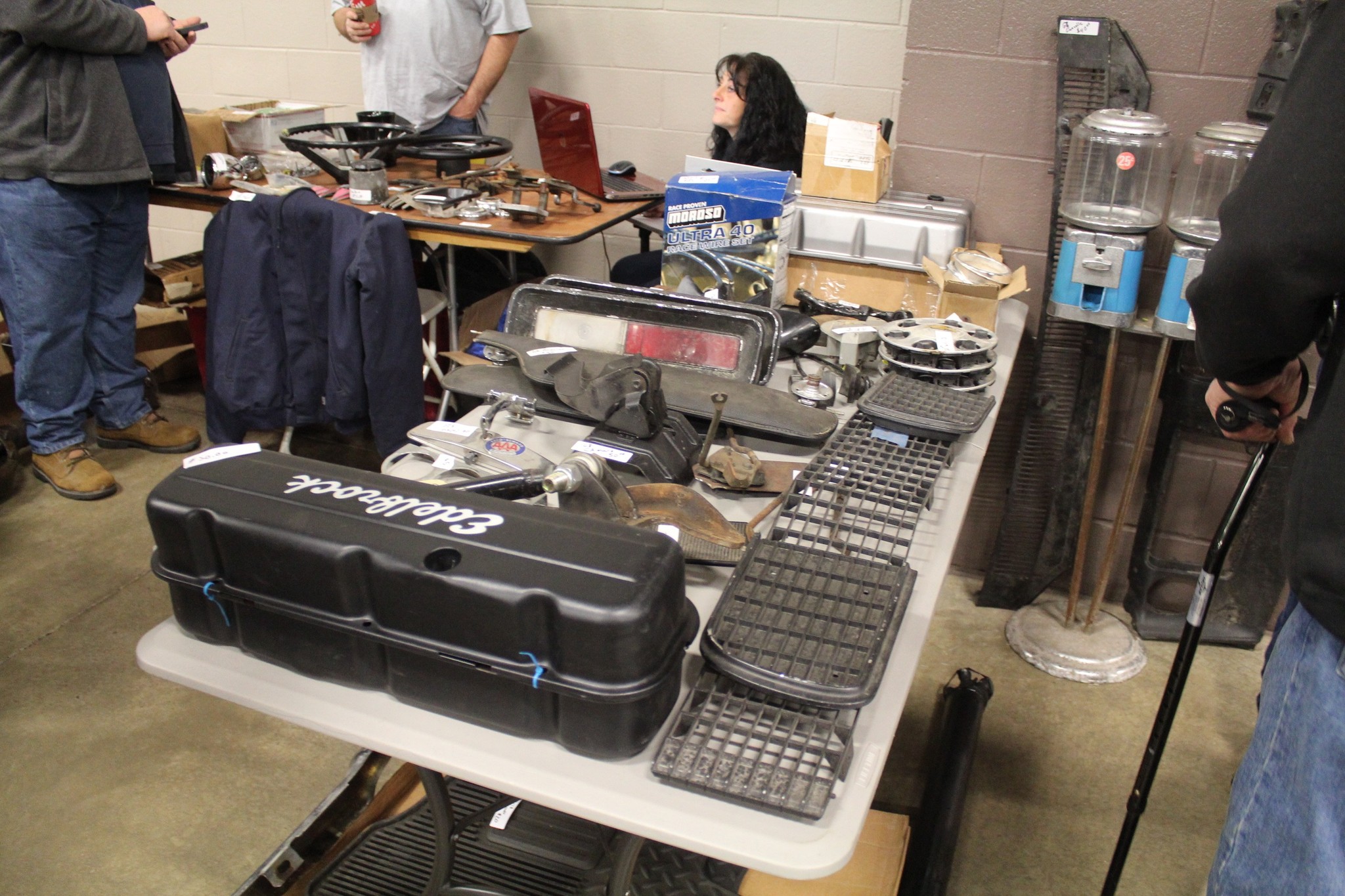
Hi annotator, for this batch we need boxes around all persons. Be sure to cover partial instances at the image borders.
[0,1,200,499]
[329,0,530,288]
[1184,0,1345,896]
[610,50,809,289]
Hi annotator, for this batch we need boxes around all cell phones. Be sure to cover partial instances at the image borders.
[175,22,209,36]
[412,187,484,204]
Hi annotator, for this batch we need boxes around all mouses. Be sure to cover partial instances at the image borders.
[608,160,636,176]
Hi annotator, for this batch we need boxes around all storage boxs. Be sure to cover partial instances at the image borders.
[219,100,346,157]
[439,277,545,370]
[801,111,893,204]
[146,435,701,762]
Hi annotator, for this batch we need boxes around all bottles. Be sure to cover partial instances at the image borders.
[349,159,388,205]
[350,1,381,35]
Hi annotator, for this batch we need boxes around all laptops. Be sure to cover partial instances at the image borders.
[528,87,668,201]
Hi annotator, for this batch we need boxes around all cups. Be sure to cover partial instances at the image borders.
[357,111,395,125]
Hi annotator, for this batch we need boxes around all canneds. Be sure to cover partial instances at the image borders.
[349,0,381,36]
[348,158,388,205]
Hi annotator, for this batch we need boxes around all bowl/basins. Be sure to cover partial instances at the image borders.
[954,253,1012,287]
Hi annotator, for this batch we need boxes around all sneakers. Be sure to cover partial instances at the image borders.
[95,410,202,453]
[31,441,118,499]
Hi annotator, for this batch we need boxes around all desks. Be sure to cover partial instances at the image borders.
[152,151,667,399]
[137,278,1029,878]
